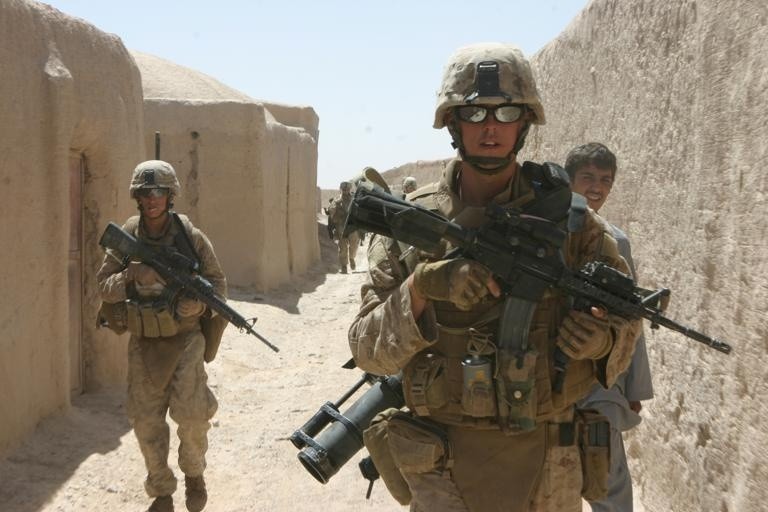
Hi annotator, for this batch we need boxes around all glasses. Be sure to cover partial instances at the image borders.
[452,105,524,124]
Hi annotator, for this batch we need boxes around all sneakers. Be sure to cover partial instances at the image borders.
[145,494,174,512]
[185,473,207,512]
[340,258,356,274]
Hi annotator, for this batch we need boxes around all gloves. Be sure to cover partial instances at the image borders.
[414,257,492,307]
[175,296,206,318]
[557,302,613,361]
[127,261,166,290]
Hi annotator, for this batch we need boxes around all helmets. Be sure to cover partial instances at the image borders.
[340,182,352,190]
[403,177,417,193]
[434,38,546,129]
[130,159,180,199]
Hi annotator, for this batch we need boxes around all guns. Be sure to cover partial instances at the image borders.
[340,184,730,353]
[98,222,279,353]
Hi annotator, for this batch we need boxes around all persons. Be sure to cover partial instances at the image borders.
[95,158,228,511]
[347,42,645,511]
[323,174,419,277]
[563,141,655,510]
